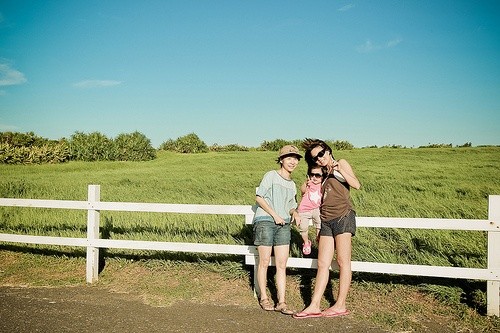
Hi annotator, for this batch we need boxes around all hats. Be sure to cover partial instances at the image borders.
[279,144,303,158]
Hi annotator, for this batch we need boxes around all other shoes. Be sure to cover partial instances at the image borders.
[275,302,292,314]
[259,297,275,310]
[303,239,311,254]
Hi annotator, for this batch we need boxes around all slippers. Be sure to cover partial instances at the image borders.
[321,308,350,317]
[292,309,322,318]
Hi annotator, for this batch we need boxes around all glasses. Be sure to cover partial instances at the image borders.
[312,148,325,161]
[310,172,323,177]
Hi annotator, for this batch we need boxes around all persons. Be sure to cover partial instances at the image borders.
[292,138,361,318]
[253,146,300,314]
[296,164,328,255]
